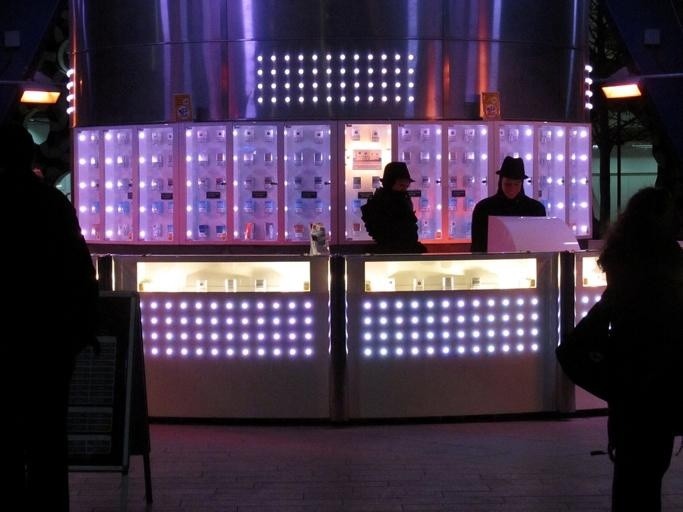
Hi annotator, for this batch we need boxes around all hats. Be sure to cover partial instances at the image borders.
[379,162,414,183]
[495,156,529,180]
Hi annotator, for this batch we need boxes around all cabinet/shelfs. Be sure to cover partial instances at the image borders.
[341,121,594,245]
[344,246,565,419]
[71,120,340,258]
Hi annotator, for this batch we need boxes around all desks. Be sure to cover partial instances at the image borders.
[127,251,335,421]
[563,250,608,328]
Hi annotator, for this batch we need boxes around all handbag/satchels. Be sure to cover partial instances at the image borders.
[555,289,618,401]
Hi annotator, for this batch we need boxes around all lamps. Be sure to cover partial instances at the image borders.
[0,70,61,105]
[602,65,683,100]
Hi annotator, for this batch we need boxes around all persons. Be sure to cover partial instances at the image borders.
[0,122,101,512]
[359,162,418,250]
[470,156,546,250]
[555,120,682,512]
